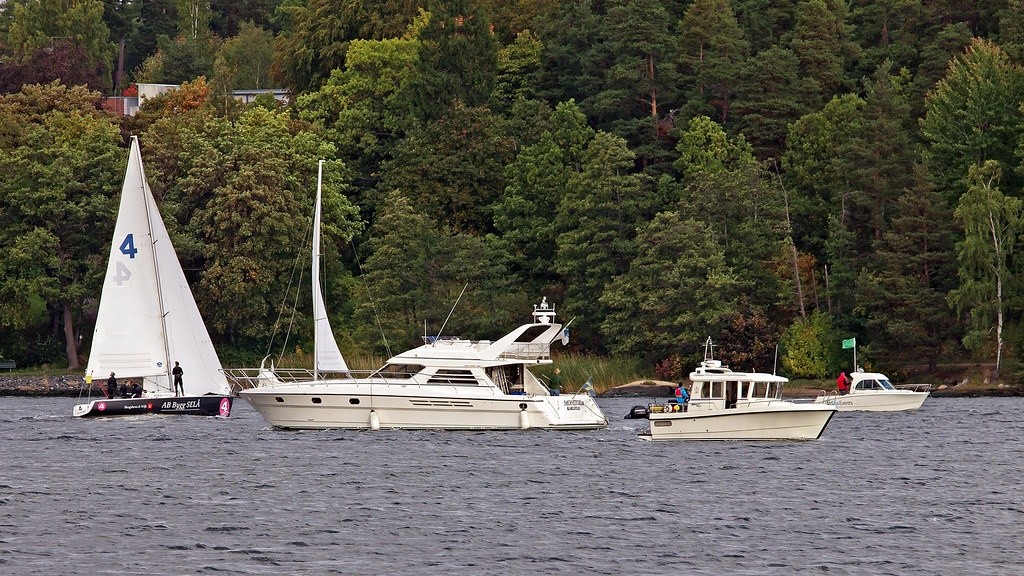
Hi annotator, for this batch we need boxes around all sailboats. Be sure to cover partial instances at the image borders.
[73,137,237,418]
[258,159,394,388]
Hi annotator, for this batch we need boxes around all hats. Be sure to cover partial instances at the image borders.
[554,368,561,374]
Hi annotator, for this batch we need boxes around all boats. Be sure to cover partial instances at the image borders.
[650,336,836,441]
[218,297,609,431]
[813,369,932,412]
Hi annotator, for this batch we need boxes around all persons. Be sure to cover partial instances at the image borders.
[836,371,852,395]
[107,372,117,399]
[549,367,563,396]
[172,362,185,397]
[120,379,142,399]
[675,382,689,412]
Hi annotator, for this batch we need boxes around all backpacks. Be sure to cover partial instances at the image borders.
[674,388,683,398]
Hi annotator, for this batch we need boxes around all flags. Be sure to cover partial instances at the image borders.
[841,337,856,349]
[584,378,596,397]
[553,322,570,346]
